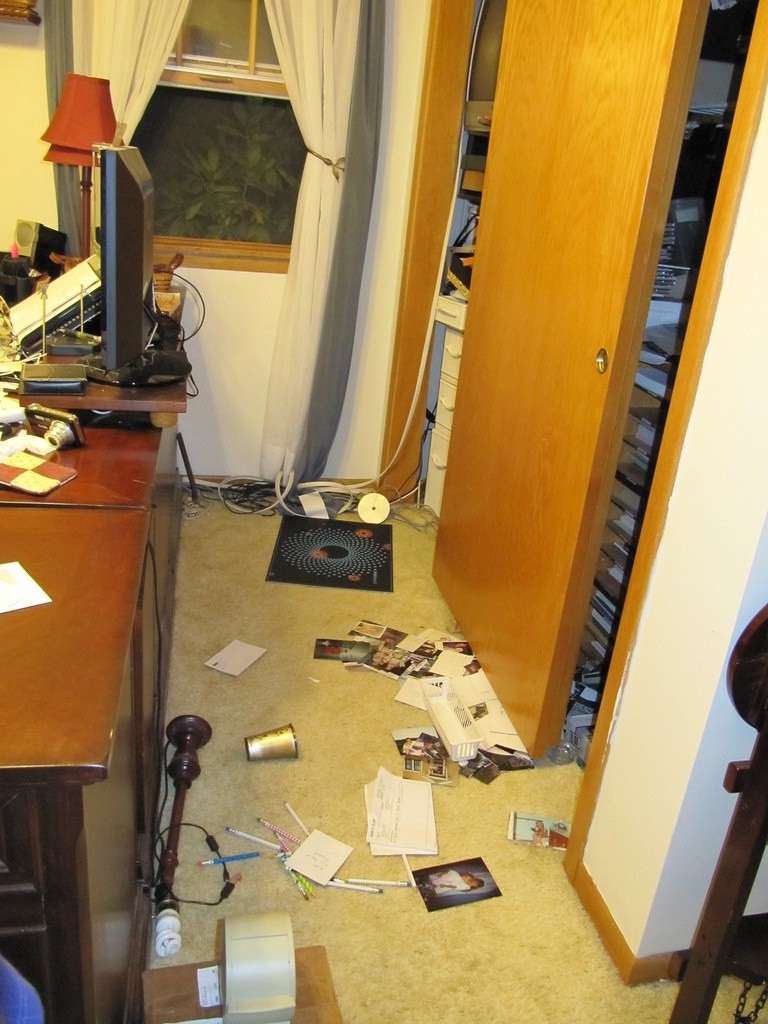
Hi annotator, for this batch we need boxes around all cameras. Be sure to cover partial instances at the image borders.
[25,403,86,450]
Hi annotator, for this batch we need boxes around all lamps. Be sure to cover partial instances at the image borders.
[41,72,124,262]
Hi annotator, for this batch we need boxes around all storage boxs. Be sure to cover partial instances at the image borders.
[659,198,707,267]
[142,945,343,1024]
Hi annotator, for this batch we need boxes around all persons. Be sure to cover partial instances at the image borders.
[529,819,549,845]
[315,625,446,778]
[422,870,485,901]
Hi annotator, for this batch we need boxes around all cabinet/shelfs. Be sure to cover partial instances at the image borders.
[383,0,768,760]
[0,286,186,1024]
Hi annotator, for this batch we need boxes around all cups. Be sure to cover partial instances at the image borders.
[244,723,298,762]
[1,253,32,301]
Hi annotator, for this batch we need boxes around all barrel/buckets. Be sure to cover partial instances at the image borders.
[154,285,187,323]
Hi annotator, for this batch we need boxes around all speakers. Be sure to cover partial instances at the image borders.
[12,220,67,282]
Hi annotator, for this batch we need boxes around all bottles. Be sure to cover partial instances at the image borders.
[153,252,184,292]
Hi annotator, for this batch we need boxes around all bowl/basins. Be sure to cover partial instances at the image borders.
[546,731,583,766]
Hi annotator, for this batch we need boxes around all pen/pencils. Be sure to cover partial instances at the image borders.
[226,827,288,852]
[345,878,410,887]
[285,803,310,837]
[273,832,294,856]
[331,877,345,883]
[277,855,319,901]
[257,818,304,846]
[197,852,259,866]
[326,882,383,894]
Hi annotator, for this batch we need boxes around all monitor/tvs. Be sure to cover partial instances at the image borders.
[83,146,193,387]
